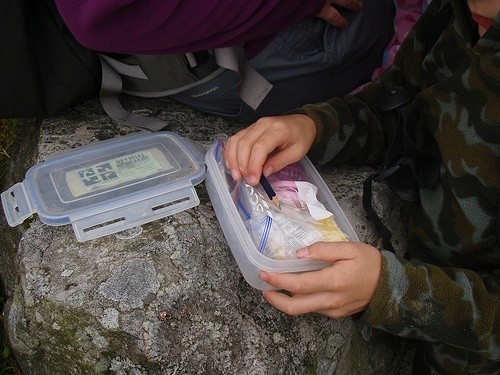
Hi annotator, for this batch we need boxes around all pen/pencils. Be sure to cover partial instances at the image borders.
[258,170,283,214]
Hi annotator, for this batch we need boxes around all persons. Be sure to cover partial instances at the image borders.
[53,0,393,122]
[223,1,500,375]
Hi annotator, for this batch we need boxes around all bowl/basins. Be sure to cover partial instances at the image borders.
[203,136,361,292]
